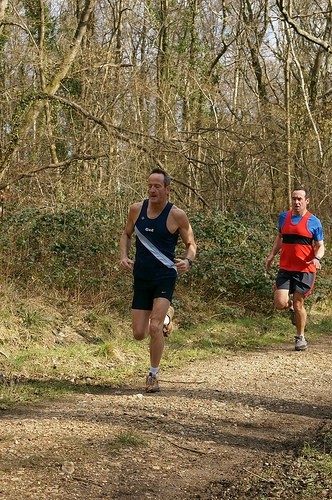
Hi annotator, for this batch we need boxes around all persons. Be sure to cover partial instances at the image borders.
[121,169,196,394]
[266,189,324,348]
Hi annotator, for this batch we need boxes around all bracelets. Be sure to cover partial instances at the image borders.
[185,257,192,269]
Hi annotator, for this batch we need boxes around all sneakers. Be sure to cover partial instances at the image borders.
[290,309,296,326]
[146,372,160,392]
[162,306,174,338]
[294,336,308,350]
